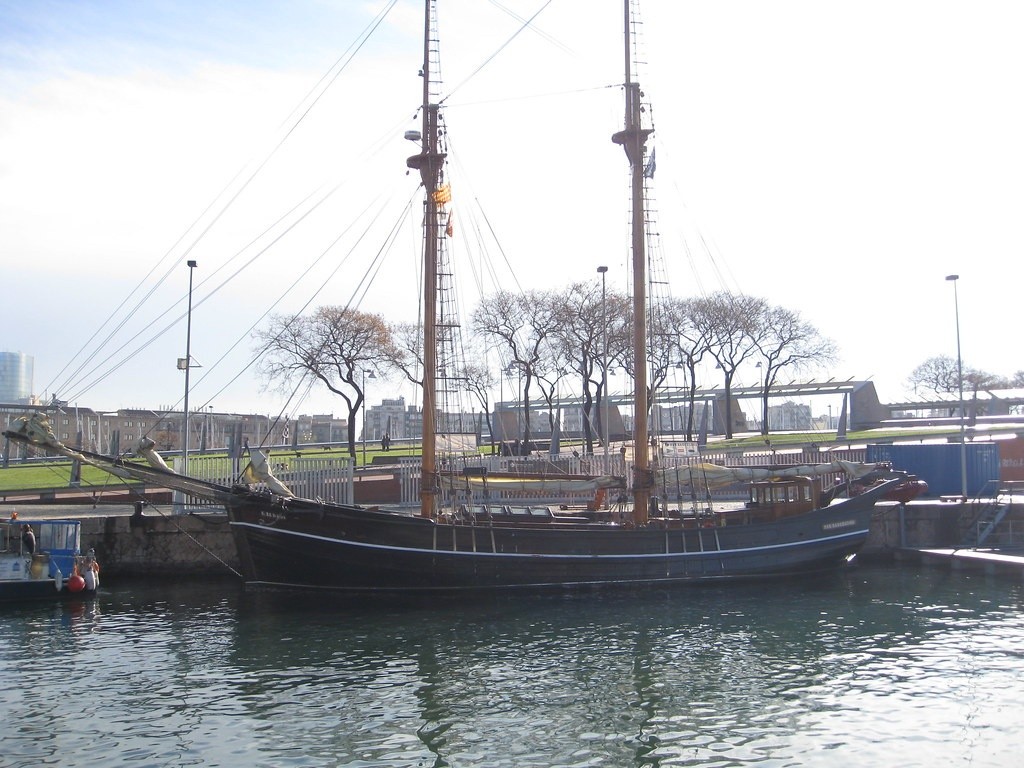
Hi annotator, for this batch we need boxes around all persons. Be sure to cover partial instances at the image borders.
[381,433,391,452]
[498,441,509,456]
[240,437,250,456]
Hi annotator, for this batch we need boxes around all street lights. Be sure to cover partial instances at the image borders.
[180,259,199,510]
[362,369,377,471]
[597,265,609,510]
[500,367,514,467]
[556,367,568,405]
[756,361,764,434]
[508,359,520,442]
[208,406,214,448]
[827,405,832,429]
[945,275,967,502]
[676,361,686,441]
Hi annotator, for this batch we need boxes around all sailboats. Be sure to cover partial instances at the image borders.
[1,0,916,612]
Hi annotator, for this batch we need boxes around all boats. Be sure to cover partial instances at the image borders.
[850,479,928,502]
[0,511,101,611]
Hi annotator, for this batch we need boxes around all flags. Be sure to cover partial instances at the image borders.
[445,209,453,237]
[643,147,656,179]
[432,182,451,208]
